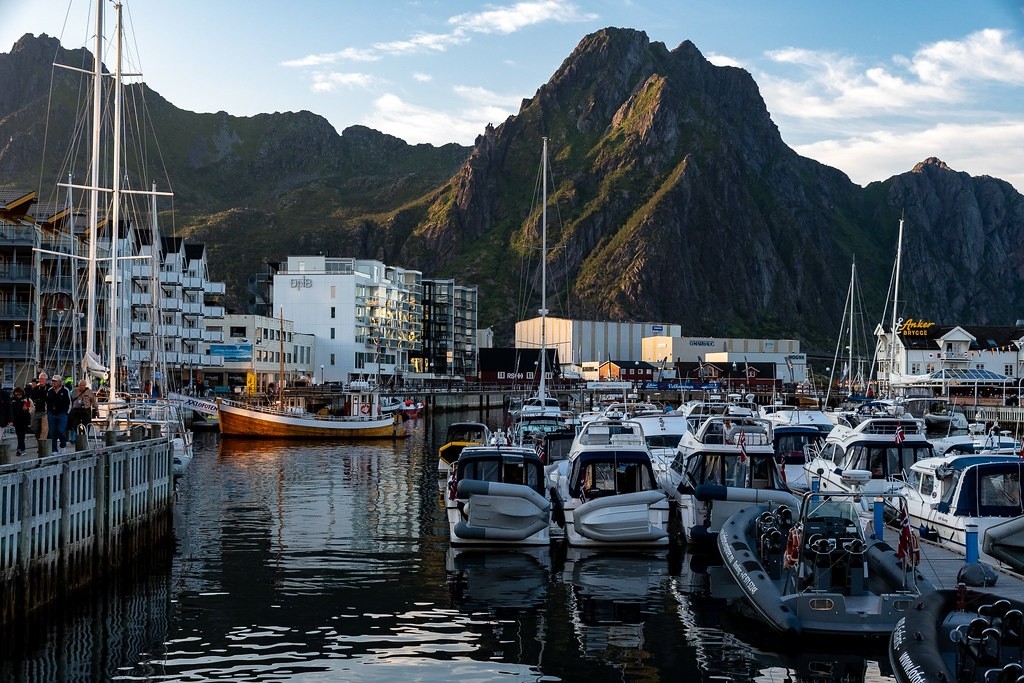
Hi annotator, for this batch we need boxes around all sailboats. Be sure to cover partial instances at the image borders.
[26,0,428,480]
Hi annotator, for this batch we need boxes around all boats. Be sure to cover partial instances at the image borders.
[436,134,1024,683]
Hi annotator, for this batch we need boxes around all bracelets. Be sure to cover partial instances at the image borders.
[96,410,100,413]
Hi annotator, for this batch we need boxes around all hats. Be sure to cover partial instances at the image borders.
[32,379,39,382]
[12,387,24,395]
[51,375,62,381]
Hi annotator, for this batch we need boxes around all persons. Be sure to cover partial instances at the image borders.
[0,372,100,456]
[722,419,738,445]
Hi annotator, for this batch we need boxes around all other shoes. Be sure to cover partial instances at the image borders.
[60,446,66,454]
[17,450,26,455]
[52,451,57,456]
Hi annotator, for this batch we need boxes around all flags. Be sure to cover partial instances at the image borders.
[928,345,1012,358]
[897,506,910,562]
[895,421,905,444]
[739,428,746,462]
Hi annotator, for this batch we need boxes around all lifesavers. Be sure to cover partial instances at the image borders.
[786,529,801,565]
[359,404,370,414]
[909,530,921,565]
